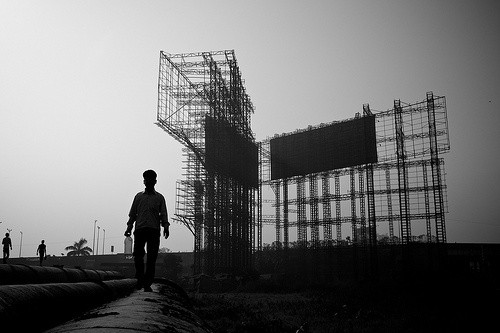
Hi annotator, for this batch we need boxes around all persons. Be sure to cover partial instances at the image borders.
[37,240,47,267]
[124,170,171,293]
[2,233,13,261]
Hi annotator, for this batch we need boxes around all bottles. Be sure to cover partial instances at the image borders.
[124,232,133,260]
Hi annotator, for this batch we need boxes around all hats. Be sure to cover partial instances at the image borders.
[142,170,157,179]
